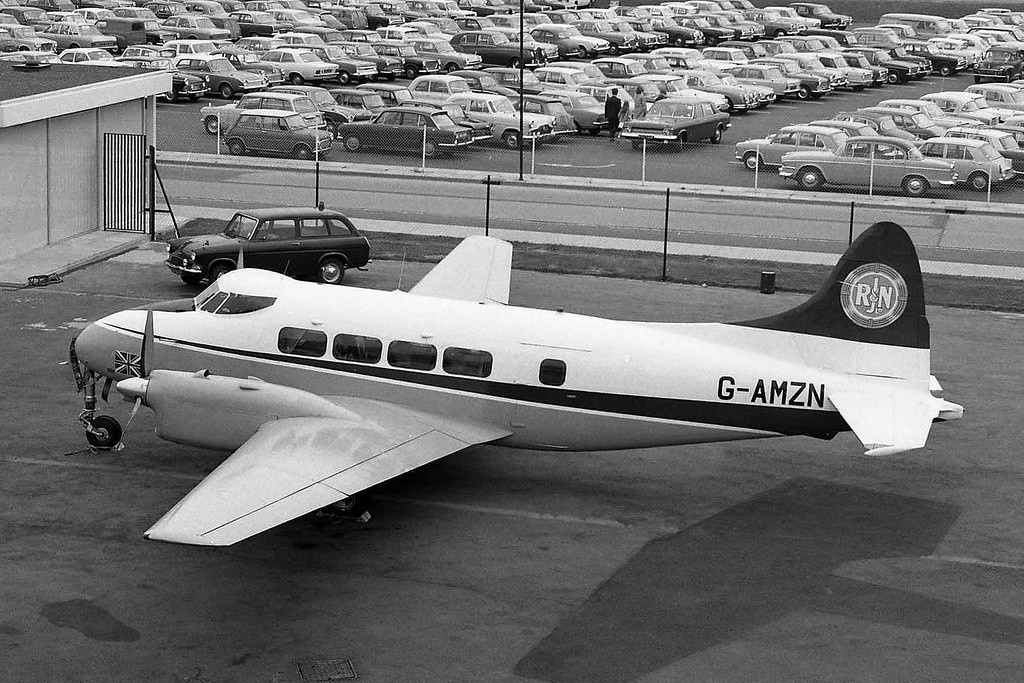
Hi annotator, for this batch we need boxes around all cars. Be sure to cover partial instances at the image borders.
[197,7,1024,160]
[735,81,1024,197]
[0,0,853,104]
[164,207,372,289]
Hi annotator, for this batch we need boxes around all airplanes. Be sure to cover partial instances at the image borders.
[69,221,968,548]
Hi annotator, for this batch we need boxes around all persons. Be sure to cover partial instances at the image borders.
[618,101,631,140]
[634,86,647,119]
[605,88,622,142]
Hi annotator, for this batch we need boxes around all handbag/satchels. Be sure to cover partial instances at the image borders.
[618,122,625,129]
